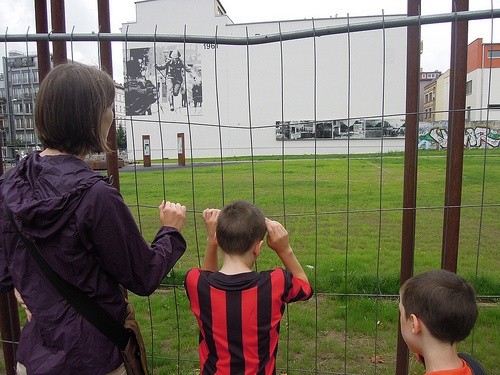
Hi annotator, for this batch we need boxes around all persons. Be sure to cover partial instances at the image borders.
[396,269,489,375]
[155,50,199,110]
[0,62,188,375]
[184,199,313,375]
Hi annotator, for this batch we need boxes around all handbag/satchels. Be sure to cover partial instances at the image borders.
[121,299,148,375]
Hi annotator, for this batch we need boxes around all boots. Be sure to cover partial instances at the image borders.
[182,93,186,107]
[169,95,174,110]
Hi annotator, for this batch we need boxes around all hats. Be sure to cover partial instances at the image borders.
[171,51,181,58]
